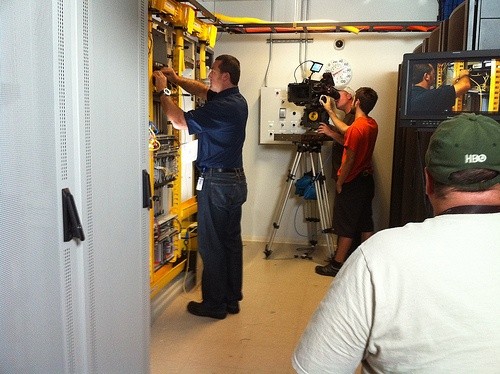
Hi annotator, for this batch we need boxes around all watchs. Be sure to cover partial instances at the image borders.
[158,87,172,97]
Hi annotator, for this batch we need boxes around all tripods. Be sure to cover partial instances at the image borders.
[263,144,335,262]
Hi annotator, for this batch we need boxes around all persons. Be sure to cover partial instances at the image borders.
[153,55,249,320]
[315,85,378,277]
[410,63,471,116]
[291,113,499,374]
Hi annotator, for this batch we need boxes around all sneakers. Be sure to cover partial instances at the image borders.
[188,301,226,319]
[226,296,238,314]
[316,263,340,277]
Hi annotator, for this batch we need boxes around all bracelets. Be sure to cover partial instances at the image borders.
[460,74,470,79]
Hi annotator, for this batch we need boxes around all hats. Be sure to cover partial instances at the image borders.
[426,111,500,191]
[335,86,355,100]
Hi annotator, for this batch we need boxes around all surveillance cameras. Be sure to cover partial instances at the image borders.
[334,38,345,50]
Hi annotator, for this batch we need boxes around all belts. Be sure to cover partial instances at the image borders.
[205,168,243,173]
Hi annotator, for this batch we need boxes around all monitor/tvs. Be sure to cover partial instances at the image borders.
[400,49,500,129]
